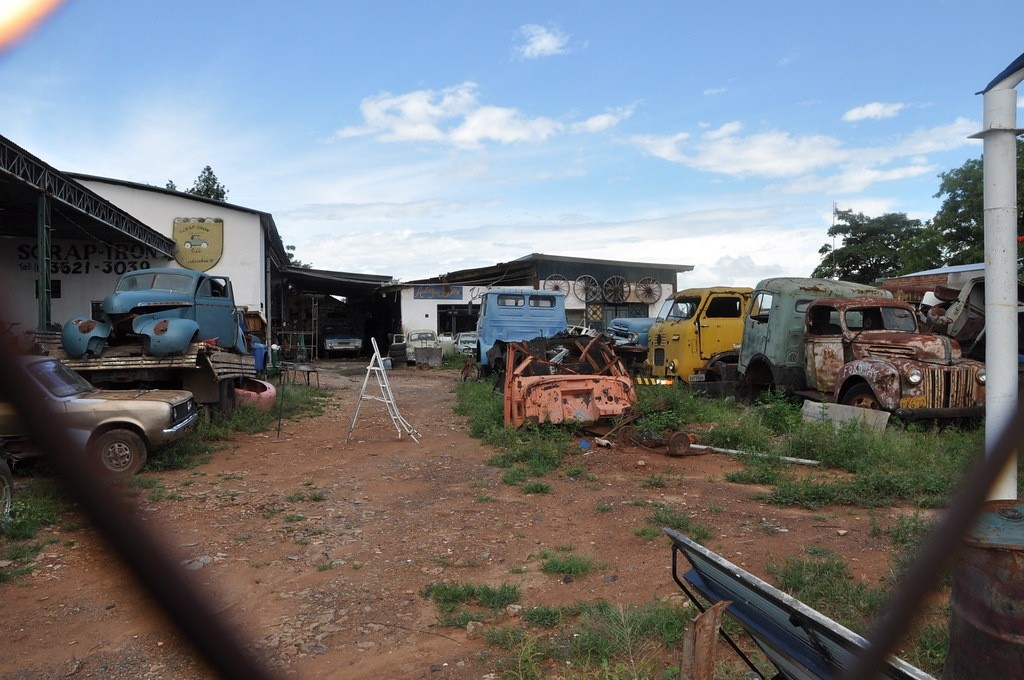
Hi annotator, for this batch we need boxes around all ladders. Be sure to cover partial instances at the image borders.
[343,336,423,444]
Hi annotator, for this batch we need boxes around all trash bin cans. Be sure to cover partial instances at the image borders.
[413,346,443,370]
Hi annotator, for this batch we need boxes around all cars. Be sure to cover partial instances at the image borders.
[392,329,454,363]
[0,349,197,479]
[317,326,363,360]
[60,267,249,354]
[452,333,477,358]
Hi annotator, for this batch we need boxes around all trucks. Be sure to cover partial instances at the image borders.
[746,298,985,423]
[648,286,760,382]
[23,310,270,426]
